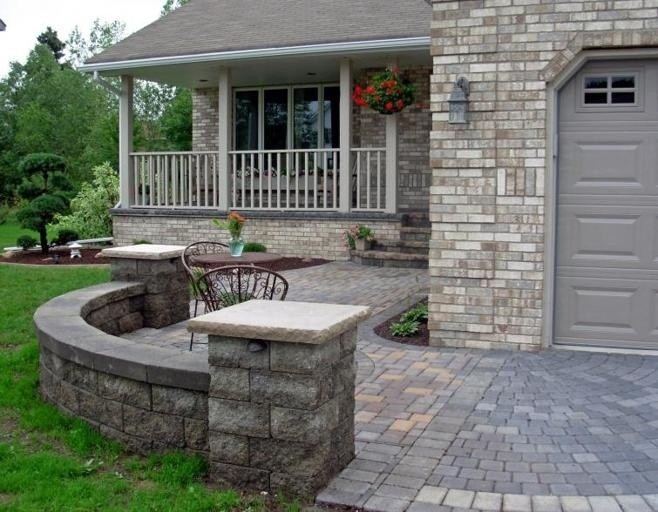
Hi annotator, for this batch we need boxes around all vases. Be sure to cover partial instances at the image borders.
[355,238,372,251]
[230,239,244,256]
[379,109,393,114]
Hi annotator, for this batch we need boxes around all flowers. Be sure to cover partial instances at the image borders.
[344,225,375,249]
[353,65,414,113]
[212,213,245,239]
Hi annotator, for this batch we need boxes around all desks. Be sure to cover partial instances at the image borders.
[192,251,283,303]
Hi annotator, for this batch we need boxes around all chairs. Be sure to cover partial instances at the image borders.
[197,265,288,311]
[182,241,229,351]
